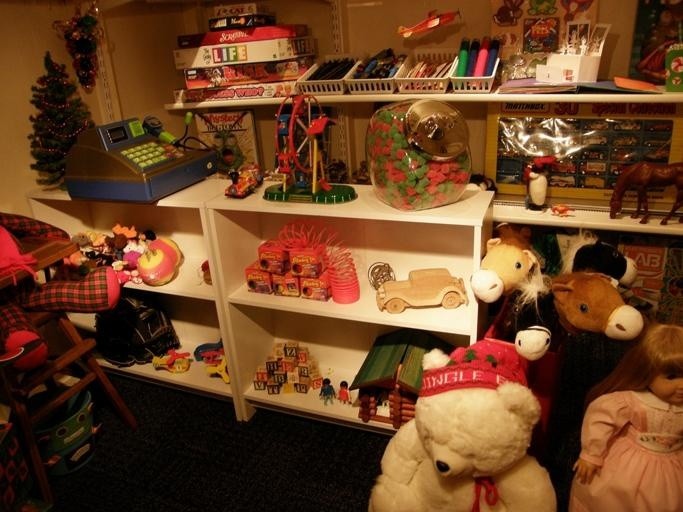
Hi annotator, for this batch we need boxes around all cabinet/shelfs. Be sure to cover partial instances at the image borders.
[26,178,242,424]
[205,178,495,435]
[163,77,683,239]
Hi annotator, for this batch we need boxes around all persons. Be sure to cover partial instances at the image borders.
[568,323,683,512]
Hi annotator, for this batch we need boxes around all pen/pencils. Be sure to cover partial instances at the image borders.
[403,56,430,78]
[359,51,406,79]
[305,56,359,91]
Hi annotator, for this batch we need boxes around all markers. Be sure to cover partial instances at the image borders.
[454,38,502,77]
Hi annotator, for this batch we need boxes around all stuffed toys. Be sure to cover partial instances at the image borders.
[368,339,558,512]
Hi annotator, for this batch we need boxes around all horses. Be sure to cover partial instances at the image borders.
[608,159,683,223]
